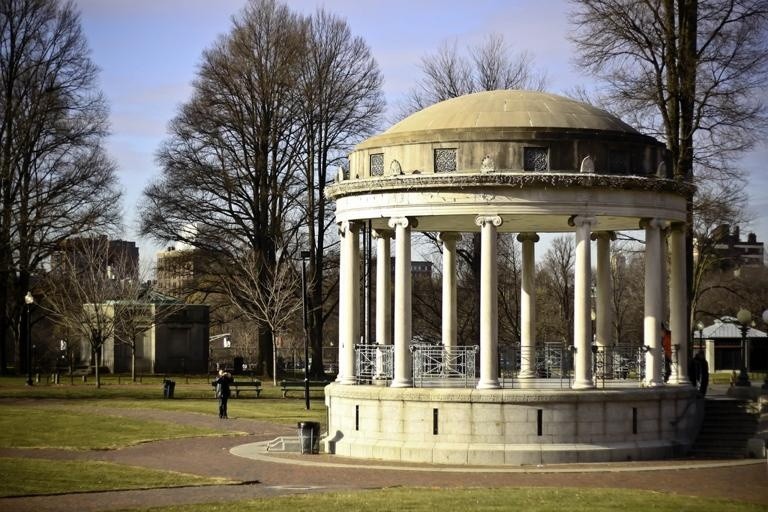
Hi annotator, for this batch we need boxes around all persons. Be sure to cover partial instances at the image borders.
[730,365,750,386]
[215,369,234,419]
[693,350,708,398]
[660,322,672,383]
[233,352,244,375]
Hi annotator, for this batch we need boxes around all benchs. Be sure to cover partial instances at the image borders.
[212,381,263,399]
[281,380,330,399]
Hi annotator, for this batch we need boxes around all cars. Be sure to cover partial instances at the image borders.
[614,366,629,374]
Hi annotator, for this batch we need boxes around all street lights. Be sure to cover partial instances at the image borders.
[24,289,34,386]
[761,309,768,392]
[697,320,704,357]
[735,308,752,386]
[296,251,310,410]
